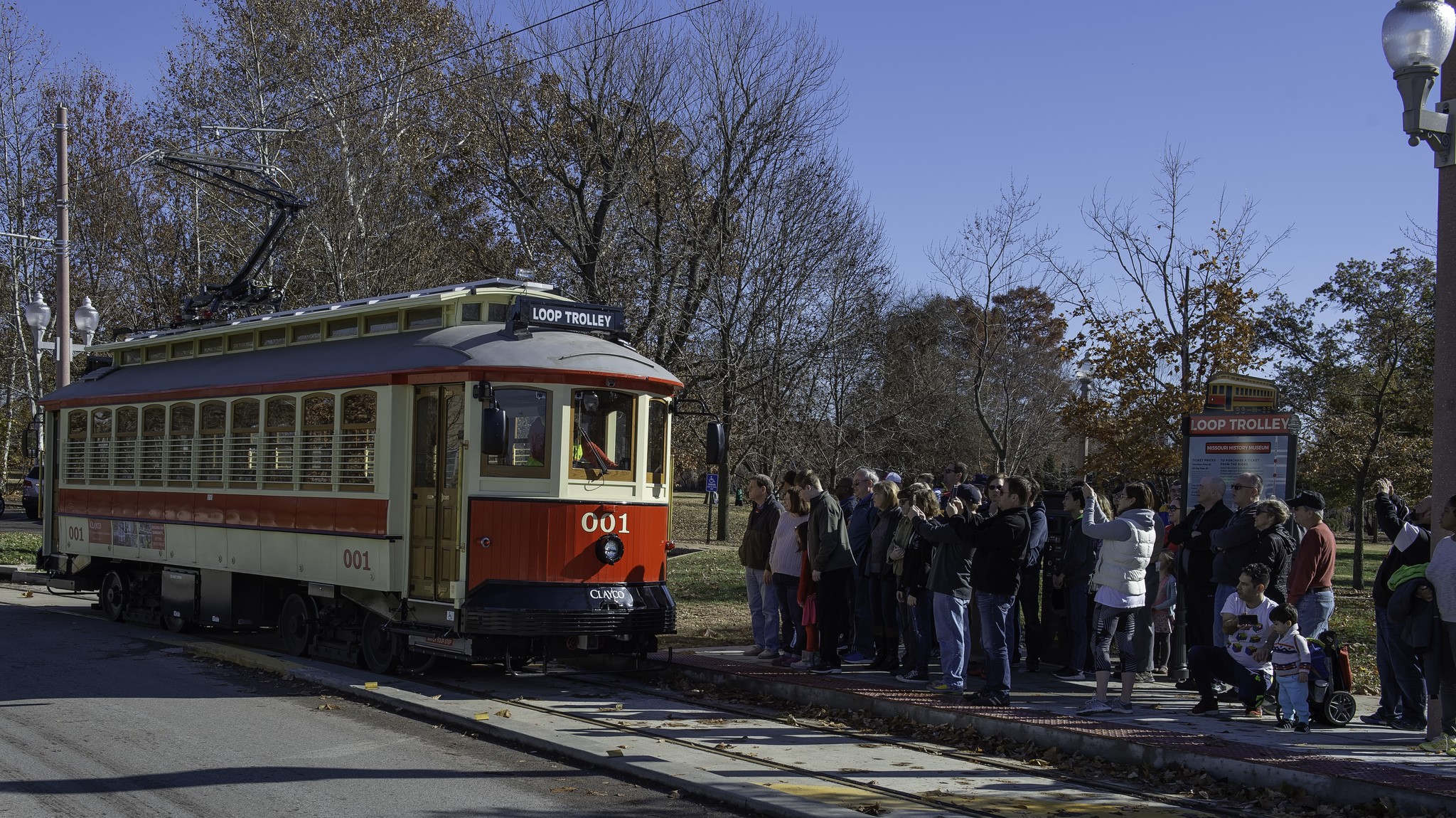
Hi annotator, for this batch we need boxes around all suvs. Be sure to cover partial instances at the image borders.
[21,461,45,520]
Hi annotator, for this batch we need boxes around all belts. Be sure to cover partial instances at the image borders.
[1307,586,1331,592]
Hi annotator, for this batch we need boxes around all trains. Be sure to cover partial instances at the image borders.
[19,141,728,687]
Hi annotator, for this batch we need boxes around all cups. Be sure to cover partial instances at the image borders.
[1314,680,1328,702]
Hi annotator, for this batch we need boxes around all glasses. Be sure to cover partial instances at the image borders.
[988,485,1002,490]
[1256,509,1268,516]
[852,480,869,485]
[1120,493,1128,499]
[1294,506,1299,511]
[1167,505,1180,512]
[796,488,803,494]
[835,485,850,489]
[1231,484,1254,490]
[1000,489,1017,496]
[1111,493,1121,498]
[780,481,787,486]
[898,499,910,505]
[945,469,956,474]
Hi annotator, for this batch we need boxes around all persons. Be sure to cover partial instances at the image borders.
[1418,494,1456,758]
[781,468,1348,691]
[943,476,1033,708]
[736,473,781,660]
[1073,472,1156,714]
[1185,562,1281,717]
[1360,478,1432,730]
[734,484,743,507]
[1252,603,1312,737]
[572,422,621,471]
[763,487,811,668]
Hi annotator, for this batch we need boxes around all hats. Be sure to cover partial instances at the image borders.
[1285,490,1325,510]
[970,474,989,485]
[956,484,980,503]
[885,472,904,485]
[783,469,803,486]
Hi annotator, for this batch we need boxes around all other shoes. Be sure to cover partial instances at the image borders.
[742,635,1168,683]
[1175,677,1284,717]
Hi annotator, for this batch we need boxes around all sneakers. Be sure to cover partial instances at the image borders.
[1360,711,1387,725]
[1293,721,1311,735]
[963,687,1010,706]
[1418,732,1456,752]
[1386,718,1424,730]
[1273,718,1295,730]
[927,680,968,694]
[1446,746,1456,756]
[1108,697,1134,714]
[1076,698,1112,715]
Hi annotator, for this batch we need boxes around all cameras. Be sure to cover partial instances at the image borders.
[1383,479,1389,486]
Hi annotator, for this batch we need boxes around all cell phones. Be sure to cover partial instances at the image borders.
[1086,473,1092,488]
[892,538,898,549]
[912,494,916,506]
[950,485,958,504]
[1238,614,1259,626]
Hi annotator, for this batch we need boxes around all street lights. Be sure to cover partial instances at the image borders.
[1370,0,1454,738]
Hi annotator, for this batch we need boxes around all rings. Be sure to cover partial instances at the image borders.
[769,578,771,580]
